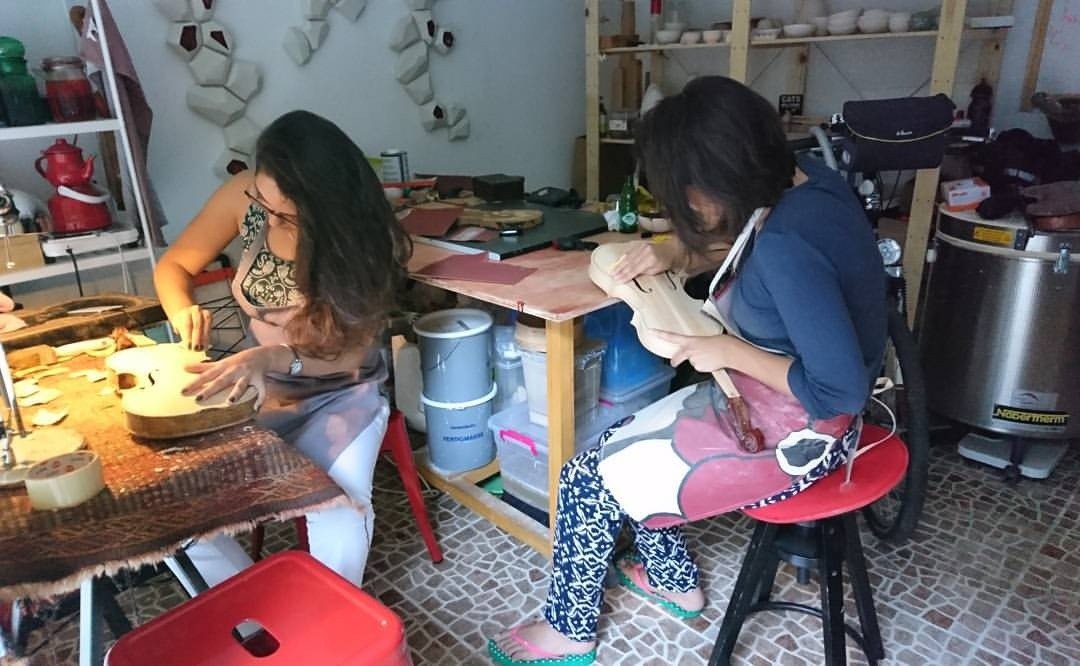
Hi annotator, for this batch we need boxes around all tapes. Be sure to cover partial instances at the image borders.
[24,450,106,512]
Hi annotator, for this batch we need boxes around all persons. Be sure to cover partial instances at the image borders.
[487,77,885,666]
[150,109,412,640]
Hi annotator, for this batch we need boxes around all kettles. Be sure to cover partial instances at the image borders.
[47,182,113,234]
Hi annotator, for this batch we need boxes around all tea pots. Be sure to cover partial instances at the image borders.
[34,138,96,187]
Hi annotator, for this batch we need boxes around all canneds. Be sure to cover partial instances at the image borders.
[380,149,410,183]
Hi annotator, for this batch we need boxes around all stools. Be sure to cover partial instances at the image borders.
[104,546,414,666]
[193,254,247,337]
[708,421,910,666]
[254,410,444,562]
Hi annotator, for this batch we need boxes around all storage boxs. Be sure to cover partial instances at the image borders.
[487,304,676,490]
[0,236,45,274]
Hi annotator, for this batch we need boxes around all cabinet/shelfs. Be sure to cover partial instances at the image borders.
[392,210,733,558]
[585,0,1054,345]
[0,0,176,342]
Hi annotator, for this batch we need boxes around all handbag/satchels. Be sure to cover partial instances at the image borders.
[841,93,953,173]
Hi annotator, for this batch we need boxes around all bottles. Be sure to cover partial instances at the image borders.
[0,36,45,126]
[608,108,639,139]
[619,175,638,233]
[599,96,609,138]
[40,55,97,122]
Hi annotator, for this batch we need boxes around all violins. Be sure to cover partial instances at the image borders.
[588,239,766,454]
[106,326,262,439]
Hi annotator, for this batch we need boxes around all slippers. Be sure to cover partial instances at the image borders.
[489,624,596,666]
[615,553,701,617]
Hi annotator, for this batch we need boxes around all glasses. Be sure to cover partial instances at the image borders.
[243,181,298,228]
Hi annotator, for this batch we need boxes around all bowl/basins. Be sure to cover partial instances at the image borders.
[638,212,674,232]
[655,22,731,44]
[749,6,942,41]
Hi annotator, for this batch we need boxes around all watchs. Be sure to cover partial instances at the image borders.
[286,346,303,376]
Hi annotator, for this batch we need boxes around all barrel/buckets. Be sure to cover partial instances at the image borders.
[917,201,1080,438]
[419,381,498,473]
[380,149,412,195]
[411,307,494,404]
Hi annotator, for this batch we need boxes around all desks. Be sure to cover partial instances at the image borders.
[0,326,366,666]
